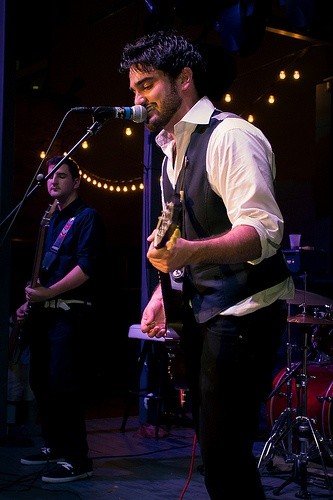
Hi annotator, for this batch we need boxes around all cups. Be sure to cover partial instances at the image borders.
[289,234,301,249]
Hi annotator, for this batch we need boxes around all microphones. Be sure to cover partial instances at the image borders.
[72,105,148,123]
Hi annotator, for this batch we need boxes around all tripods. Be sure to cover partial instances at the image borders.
[255,327,333,500]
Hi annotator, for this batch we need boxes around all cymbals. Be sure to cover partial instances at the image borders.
[286,289,332,306]
[285,314,333,325]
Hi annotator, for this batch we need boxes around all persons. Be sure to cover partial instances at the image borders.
[118,31,294,500]
[16,155,105,483]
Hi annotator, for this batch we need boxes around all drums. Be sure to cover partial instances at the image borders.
[266,361,333,459]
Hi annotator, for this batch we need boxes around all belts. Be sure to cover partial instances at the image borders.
[44,298,92,311]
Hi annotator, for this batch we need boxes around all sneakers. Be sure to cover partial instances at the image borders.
[21,447,65,464]
[41,458,94,483]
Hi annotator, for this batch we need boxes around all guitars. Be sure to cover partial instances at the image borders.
[152,202,195,415]
[16,198,59,364]
